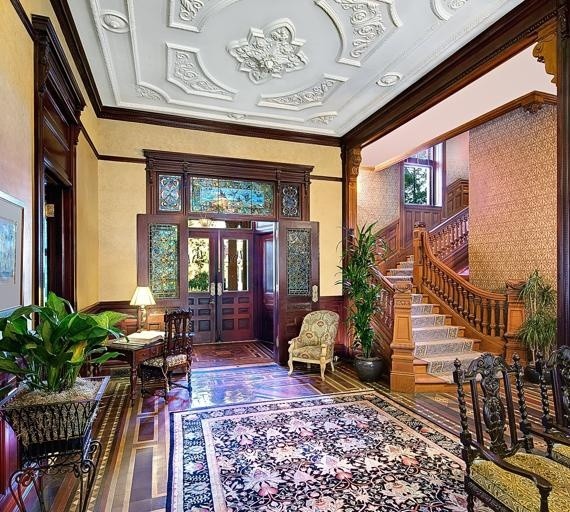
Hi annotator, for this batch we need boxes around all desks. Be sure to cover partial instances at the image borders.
[91,333,196,408]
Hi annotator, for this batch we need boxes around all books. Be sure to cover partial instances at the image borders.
[125,331,162,345]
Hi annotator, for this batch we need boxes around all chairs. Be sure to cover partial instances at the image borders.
[452,351,570,512]
[140,307,193,405]
[536,344,570,470]
[287,310,340,382]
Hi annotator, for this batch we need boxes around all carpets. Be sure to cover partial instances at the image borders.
[164,389,496,512]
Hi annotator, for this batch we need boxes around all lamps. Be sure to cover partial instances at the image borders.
[199,218,213,227]
[128,285,157,333]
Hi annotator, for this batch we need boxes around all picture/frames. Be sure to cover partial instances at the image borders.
[0,192,27,320]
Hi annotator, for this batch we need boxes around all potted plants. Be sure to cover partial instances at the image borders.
[0,290,137,460]
[333,217,395,383]
[515,269,560,384]
